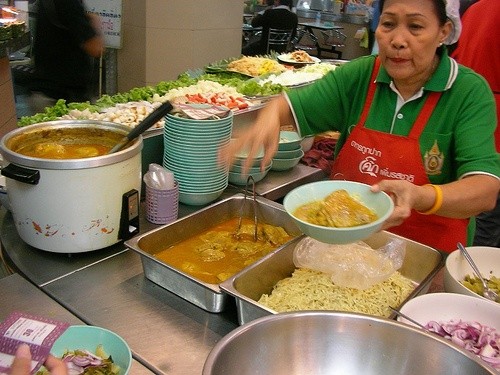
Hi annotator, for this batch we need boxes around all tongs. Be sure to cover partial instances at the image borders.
[236,175,258,241]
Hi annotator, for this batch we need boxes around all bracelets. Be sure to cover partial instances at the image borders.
[414,184,443,215]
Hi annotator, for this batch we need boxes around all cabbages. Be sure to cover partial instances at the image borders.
[300,62,340,73]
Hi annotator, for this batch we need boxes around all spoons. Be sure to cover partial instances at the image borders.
[457,242,500,301]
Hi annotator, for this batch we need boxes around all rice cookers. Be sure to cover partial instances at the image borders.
[0,119,145,255]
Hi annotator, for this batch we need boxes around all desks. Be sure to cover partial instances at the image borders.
[242,26,263,50]
[297,22,344,58]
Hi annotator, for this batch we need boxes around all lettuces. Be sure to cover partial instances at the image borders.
[198,71,293,97]
[17,71,198,127]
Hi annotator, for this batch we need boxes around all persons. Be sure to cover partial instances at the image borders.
[242,0,298,56]
[365,0,384,55]
[221,0,500,262]
[452,0,500,248]
[33,0,103,111]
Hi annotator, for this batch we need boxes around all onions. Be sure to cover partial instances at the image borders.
[63,349,103,375]
[422,319,500,373]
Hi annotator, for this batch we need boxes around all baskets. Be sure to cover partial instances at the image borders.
[145,179,179,224]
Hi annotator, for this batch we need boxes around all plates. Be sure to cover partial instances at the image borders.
[276,53,320,65]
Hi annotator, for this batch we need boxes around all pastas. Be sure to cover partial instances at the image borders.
[257,267,416,318]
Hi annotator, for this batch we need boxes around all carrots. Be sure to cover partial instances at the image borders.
[186,94,251,109]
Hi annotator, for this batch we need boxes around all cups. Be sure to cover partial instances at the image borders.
[145,180,179,224]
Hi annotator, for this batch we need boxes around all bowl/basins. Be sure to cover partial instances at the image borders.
[284,180,395,245]
[50,325,132,374]
[396,292,500,374]
[201,310,498,375]
[269,131,305,172]
[163,104,235,206]
[229,138,273,185]
[444,246,500,303]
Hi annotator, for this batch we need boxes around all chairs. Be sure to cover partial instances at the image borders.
[321,29,347,48]
[267,28,292,54]
[296,31,317,53]
